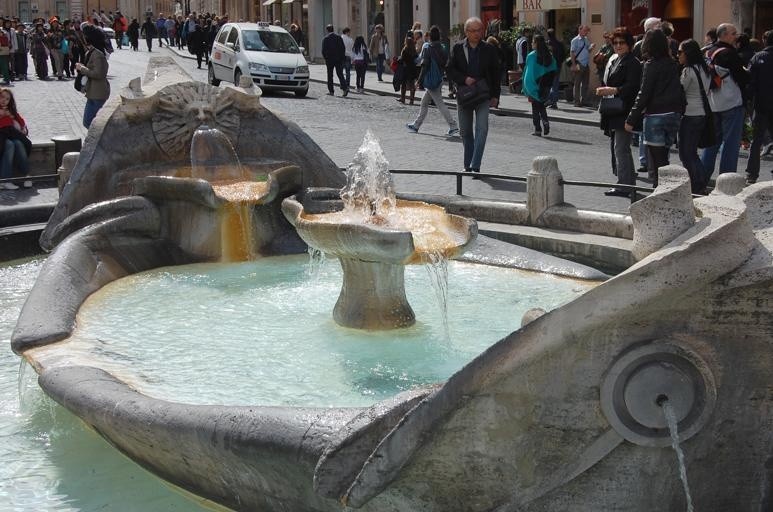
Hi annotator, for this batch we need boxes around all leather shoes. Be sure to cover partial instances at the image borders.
[395,97,407,105]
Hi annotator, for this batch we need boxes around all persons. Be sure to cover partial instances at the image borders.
[156,12,169,48]
[181,11,229,68]
[750,36,760,53]
[703,46,732,92]
[0,11,85,87]
[446,16,501,173]
[733,32,753,67]
[508,27,532,95]
[485,34,501,51]
[289,23,302,48]
[321,24,352,97]
[412,21,422,32]
[406,26,460,137]
[636,33,645,42]
[523,34,558,137]
[75,9,128,129]
[569,24,595,108]
[163,15,178,47]
[593,31,614,87]
[175,15,185,50]
[369,24,389,82]
[254,15,262,24]
[172,14,179,28]
[340,27,355,90]
[595,27,643,197]
[413,29,425,55]
[352,36,369,94]
[743,29,773,184]
[424,31,430,43]
[238,18,245,23]
[631,16,664,61]
[544,27,566,109]
[0,88,33,190]
[395,36,419,106]
[662,20,679,58]
[700,23,748,176]
[404,29,415,42]
[140,16,156,52]
[273,19,283,27]
[699,27,718,61]
[125,18,140,52]
[676,38,712,195]
[414,42,432,65]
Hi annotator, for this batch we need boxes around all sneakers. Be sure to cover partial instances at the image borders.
[637,165,649,173]
[342,88,350,97]
[353,87,366,94]
[326,90,335,96]
[0,182,19,191]
[542,120,550,135]
[23,175,34,188]
[745,175,756,187]
[605,187,632,197]
[446,128,462,137]
[531,130,544,136]
[405,122,419,134]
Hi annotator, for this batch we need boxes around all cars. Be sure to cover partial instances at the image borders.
[207,23,310,97]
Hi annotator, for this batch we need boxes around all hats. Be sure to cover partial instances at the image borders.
[47,15,59,23]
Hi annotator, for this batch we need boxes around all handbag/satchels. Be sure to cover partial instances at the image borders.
[73,47,98,93]
[457,77,493,111]
[362,47,374,67]
[422,43,441,89]
[565,41,587,68]
[598,85,626,117]
[383,38,392,62]
[687,65,718,153]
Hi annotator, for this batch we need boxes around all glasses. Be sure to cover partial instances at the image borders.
[676,49,685,56]
[611,39,628,46]
[466,26,483,34]
